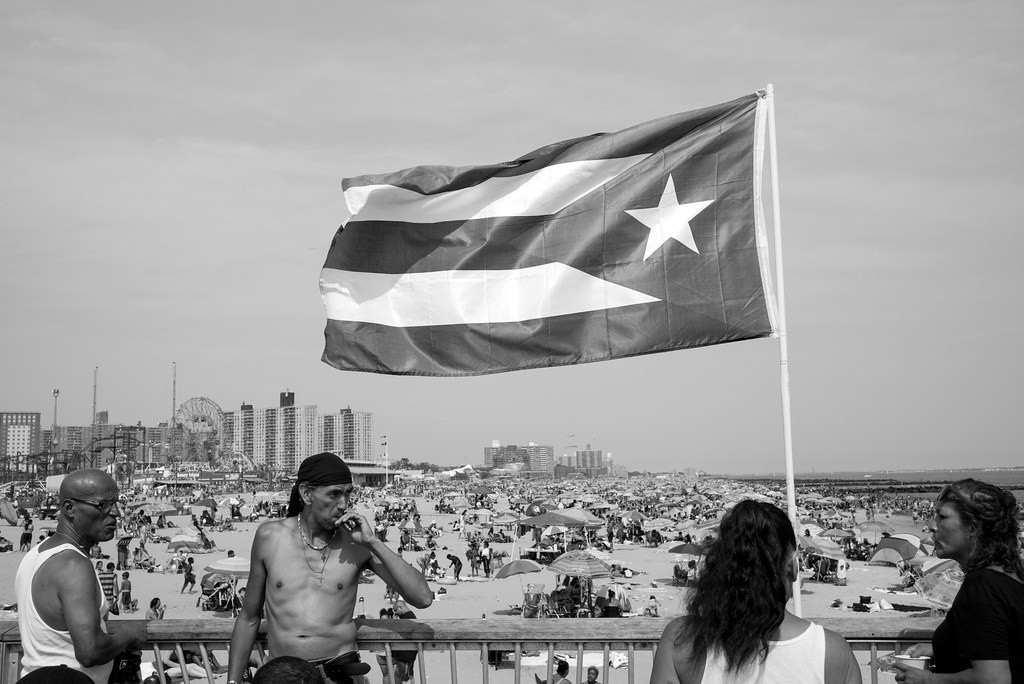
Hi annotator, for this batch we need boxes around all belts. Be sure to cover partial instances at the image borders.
[314,651,371,678]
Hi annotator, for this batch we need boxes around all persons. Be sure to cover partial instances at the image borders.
[227,452,433,684]
[0,477,935,684]
[649,500,864,684]
[15,468,147,684]
[892,478,1024,684]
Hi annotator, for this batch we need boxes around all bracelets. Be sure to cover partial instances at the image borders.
[227,680,238,684]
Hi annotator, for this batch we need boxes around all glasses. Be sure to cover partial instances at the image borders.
[69,498,124,510]
[937,485,981,519]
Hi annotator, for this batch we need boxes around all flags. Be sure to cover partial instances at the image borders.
[319,88,781,376]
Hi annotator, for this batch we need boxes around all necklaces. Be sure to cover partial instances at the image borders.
[297,513,339,550]
[300,512,336,582]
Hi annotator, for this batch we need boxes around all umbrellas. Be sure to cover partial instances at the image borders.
[619,510,648,529]
[800,535,846,580]
[641,517,676,532]
[548,549,614,579]
[492,558,544,582]
[856,521,895,542]
[913,560,966,609]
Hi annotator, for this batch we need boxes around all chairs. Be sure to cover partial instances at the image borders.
[523,583,546,619]
